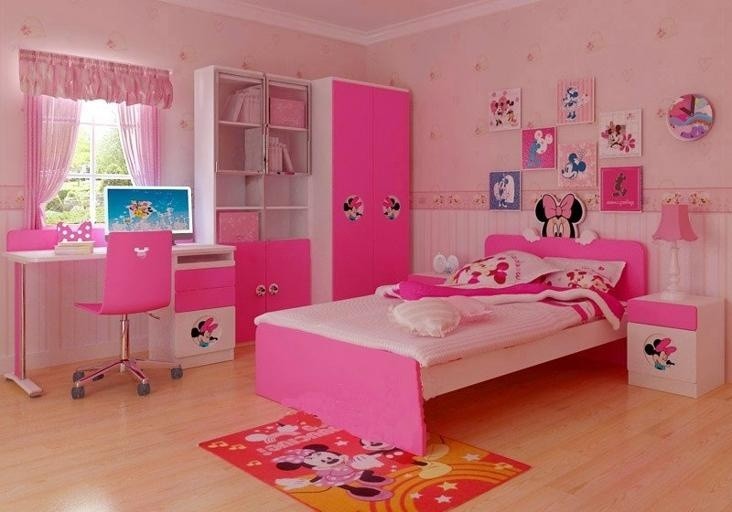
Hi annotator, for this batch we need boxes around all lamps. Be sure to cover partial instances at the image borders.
[652,204,698,300]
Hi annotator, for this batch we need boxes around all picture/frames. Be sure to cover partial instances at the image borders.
[557,141,598,188]
[555,78,595,126]
[600,166,642,211]
[488,87,521,131]
[520,126,557,171]
[488,170,522,212]
[598,108,643,158]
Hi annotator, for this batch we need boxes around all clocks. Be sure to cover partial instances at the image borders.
[666,93,715,142]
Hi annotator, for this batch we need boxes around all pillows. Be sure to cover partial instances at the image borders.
[435,249,626,293]
[389,295,492,337]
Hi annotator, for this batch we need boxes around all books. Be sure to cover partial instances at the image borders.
[54,241,97,254]
[221,85,262,125]
[268,136,295,172]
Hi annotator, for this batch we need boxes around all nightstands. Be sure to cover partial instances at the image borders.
[408,273,452,284]
[627,293,726,398]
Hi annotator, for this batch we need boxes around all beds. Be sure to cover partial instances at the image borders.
[254,234,648,456]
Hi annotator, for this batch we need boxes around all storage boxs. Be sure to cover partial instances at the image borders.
[270,98,305,128]
[216,213,259,245]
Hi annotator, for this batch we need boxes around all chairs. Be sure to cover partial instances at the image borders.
[71,230,183,398]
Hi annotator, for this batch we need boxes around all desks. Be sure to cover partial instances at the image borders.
[2,228,236,396]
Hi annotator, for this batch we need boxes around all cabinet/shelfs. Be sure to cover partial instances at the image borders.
[312,76,410,304]
[194,66,311,346]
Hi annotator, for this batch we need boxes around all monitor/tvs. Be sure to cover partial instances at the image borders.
[104,186,193,245]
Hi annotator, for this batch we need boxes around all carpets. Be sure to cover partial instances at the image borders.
[199,407,531,512]
